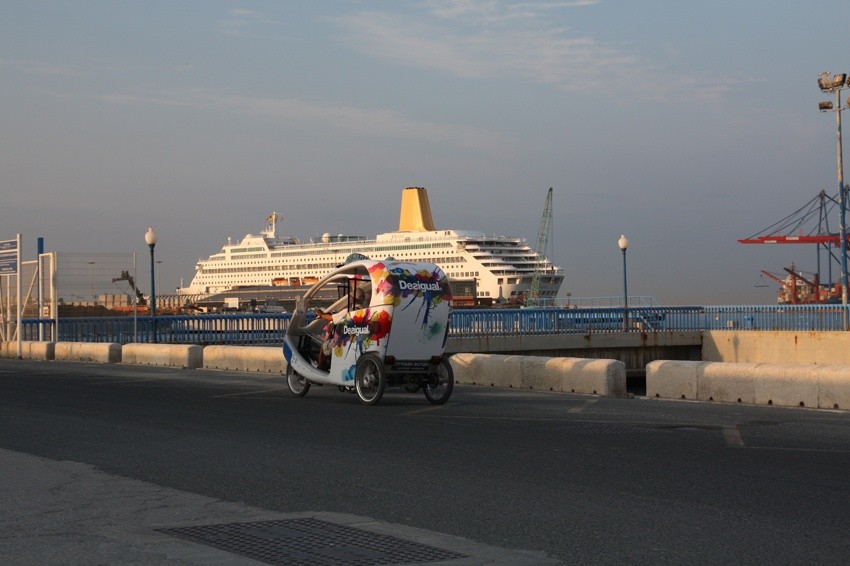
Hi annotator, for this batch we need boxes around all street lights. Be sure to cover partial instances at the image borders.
[144,226,158,344]
[616,234,630,333]
[817,72,850,332]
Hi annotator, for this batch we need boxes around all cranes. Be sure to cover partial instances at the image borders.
[737,188,850,306]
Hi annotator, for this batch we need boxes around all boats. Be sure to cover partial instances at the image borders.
[304,277,318,286]
[272,279,288,287]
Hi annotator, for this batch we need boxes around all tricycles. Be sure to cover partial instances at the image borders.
[280,258,454,407]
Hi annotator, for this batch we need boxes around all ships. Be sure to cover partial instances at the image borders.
[176,186,566,334]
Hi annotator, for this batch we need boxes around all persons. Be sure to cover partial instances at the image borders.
[314,287,366,373]
